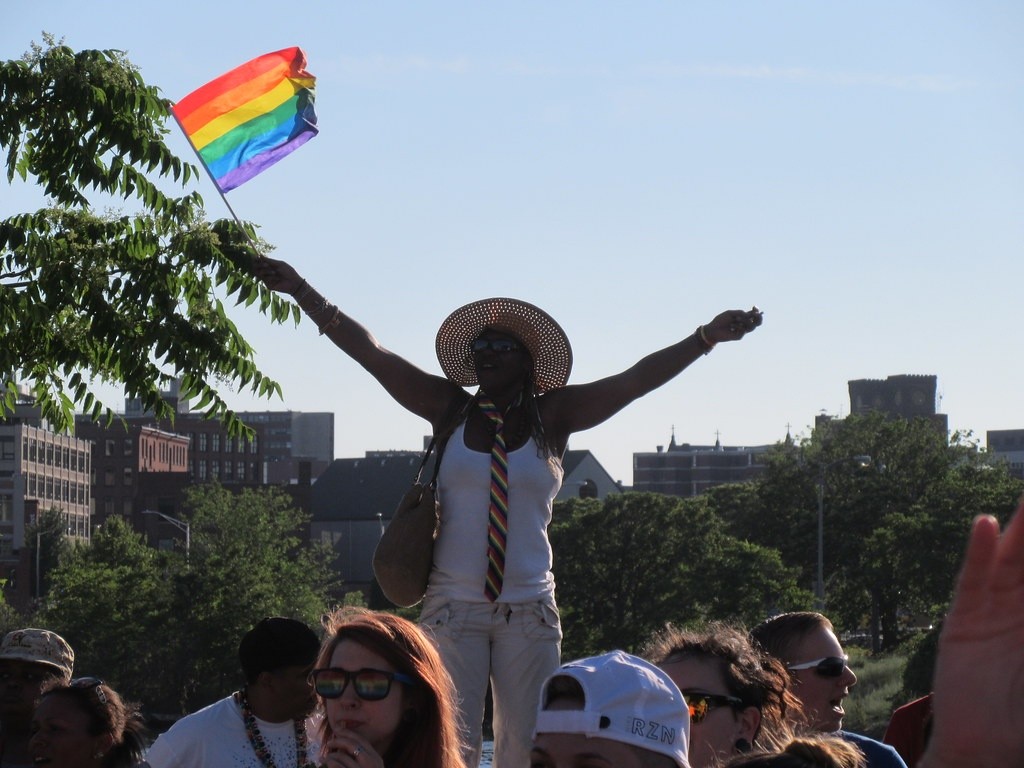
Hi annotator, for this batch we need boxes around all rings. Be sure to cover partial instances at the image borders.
[351,745,364,758]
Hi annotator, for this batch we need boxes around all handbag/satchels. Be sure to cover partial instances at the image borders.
[371,397,464,608]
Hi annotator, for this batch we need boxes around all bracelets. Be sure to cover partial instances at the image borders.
[695,325,714,355]
[291,279,305,296]
[296,288,341,336]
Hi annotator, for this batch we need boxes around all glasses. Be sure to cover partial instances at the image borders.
[682,690,742,726]
[789,656,851,679]
[41,677,115,727]
[472,338,525,355]
[312,667,408,702]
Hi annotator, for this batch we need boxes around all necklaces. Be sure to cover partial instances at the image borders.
[480,401,527,449]
[237,685,309,768]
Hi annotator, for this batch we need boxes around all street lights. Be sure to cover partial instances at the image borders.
[818,453,871,620]
[35,529,53,599]
[141,510,190,571]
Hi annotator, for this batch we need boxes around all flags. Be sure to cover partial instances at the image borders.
[171,46,319,193]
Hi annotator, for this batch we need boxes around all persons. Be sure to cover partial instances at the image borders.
[0,626,74,768]
[24,677,151,768]
[146,616,323,767]
[307,604,471,768]
[643,618,864,768]
[251,253,763,768]
[529,648,694,768]
[748,610,909,767]
[883,498,1024,768]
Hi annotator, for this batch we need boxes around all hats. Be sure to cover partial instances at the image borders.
[0,629,74,682]
[435,298,572,392]
[533,651,696,768]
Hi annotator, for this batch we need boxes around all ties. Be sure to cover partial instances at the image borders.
[480,397,521,605]
